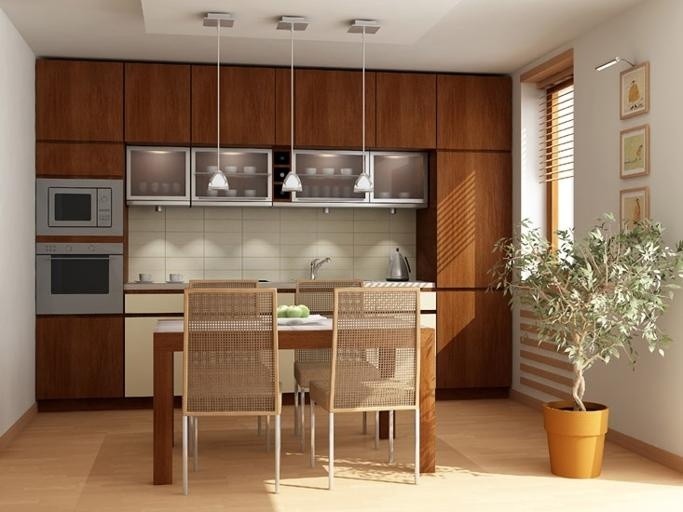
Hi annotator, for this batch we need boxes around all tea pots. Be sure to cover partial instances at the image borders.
[386,248,410,282]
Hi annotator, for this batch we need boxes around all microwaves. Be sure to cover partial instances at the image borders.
[34,178,124,237]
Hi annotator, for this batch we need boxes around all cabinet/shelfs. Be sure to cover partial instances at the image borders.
[273,150,292,203]
[35,315,124,413]
[415,151,512,287]
[437,290,510,393]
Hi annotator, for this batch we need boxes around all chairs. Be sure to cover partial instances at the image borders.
[294,278,380,452]
[311,288,421,491]
[182,286,284,494]
[181,278,271,450]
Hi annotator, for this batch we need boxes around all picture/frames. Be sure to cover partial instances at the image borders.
[619,186,652,236]
[619,62,650,120]
[618,125,651,179]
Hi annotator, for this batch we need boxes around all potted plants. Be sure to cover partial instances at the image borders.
[486,211,683,478]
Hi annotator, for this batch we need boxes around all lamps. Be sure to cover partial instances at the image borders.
[275,16,312,190]
[202,13,235,189]
[595,57,635,72]
[348,19,382,194]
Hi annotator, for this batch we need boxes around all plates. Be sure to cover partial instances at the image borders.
[134,280,154,283]
[260,314,326,326]
[165,280,184,284]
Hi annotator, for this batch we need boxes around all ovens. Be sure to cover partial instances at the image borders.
[34,255,122,314]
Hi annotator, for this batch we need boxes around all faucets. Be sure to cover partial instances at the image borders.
[311,256,331,280]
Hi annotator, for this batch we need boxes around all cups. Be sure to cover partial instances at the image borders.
[379,192,391,198]
[205,166,256,196]
[139,273,150,280]
[300,168,353,198]
[137,181,183,194]
[169,273,179,280]
[396,192,410,198]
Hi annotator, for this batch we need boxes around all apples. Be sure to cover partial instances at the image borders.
[277,304,309,318]
